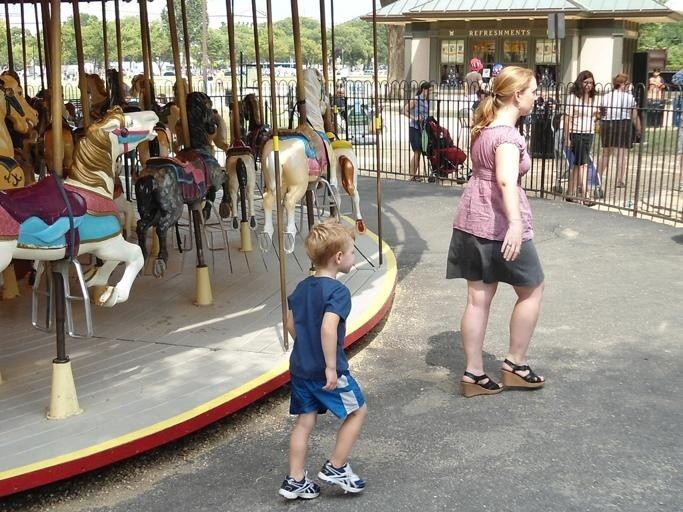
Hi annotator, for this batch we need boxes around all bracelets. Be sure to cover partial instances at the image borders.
[507,217,523,223]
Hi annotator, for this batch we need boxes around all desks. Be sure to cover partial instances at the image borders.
[518,106,552,140]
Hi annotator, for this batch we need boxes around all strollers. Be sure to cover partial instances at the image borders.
[417,112,474,184]
[546,108,604,201]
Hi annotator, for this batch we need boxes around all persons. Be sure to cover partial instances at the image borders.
[400,82,433,181]
[530,67,683,207]
[277,218,370,502]
[444,66,547,398]
[333,88,350,118]
[464,58,503,127]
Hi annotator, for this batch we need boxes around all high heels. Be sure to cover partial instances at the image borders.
[500,359,546,387]
[461,371,504,397]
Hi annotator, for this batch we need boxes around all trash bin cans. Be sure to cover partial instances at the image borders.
[530,106,555,159]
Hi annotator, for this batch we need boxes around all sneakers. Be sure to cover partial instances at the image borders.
[278,471,320,500]
[317,459,366,494]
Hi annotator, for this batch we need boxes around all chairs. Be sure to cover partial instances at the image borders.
[457,108,476,148]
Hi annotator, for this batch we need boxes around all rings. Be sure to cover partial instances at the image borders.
[507,242,513,248]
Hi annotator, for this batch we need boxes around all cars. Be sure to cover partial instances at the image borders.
[181,63,196,76]
[27,61,176,79]
